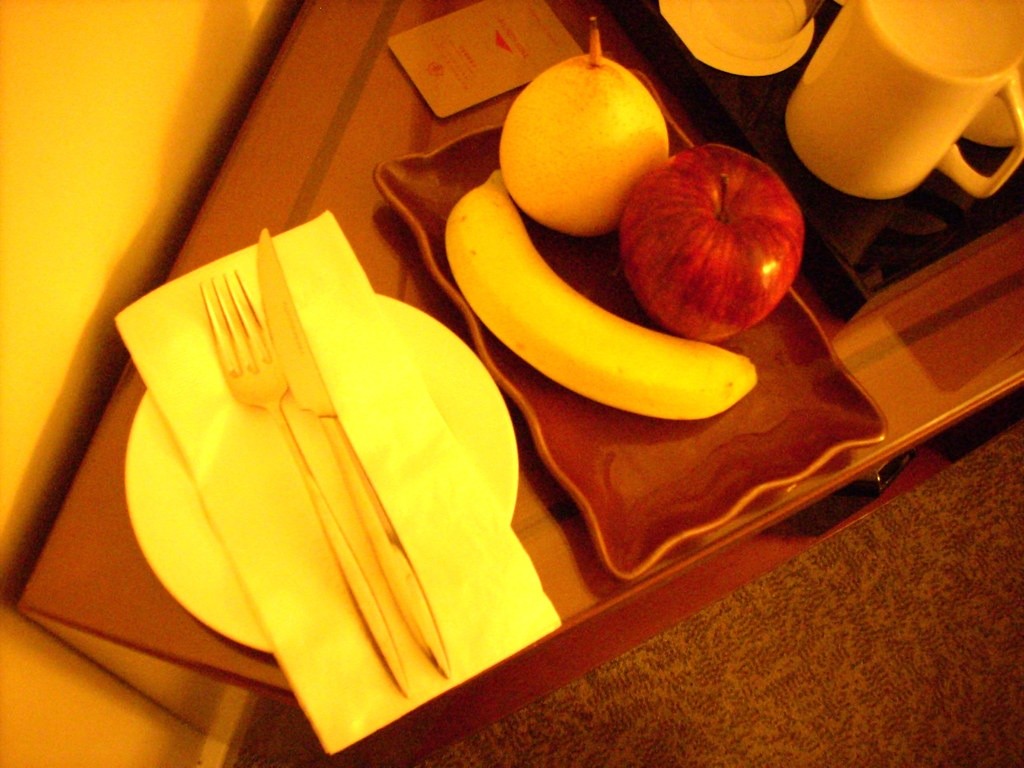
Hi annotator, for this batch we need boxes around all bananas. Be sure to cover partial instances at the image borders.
[445,169,757,421]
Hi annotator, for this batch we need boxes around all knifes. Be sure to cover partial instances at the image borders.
[256,227,452,681]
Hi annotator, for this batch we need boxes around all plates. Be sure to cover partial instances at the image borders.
[123,295,520,653]
[372,68,890,582]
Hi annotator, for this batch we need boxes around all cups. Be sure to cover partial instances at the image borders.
[784,0,1024,198]
[690,0,824,59]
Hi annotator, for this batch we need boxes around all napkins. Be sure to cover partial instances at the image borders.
[114,209,561,757]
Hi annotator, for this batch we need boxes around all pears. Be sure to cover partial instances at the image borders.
[499,14,668,235]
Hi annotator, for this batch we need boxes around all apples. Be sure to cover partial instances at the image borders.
[620,143,805,345]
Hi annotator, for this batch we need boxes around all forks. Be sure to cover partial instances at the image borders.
[201,268,414,700]
